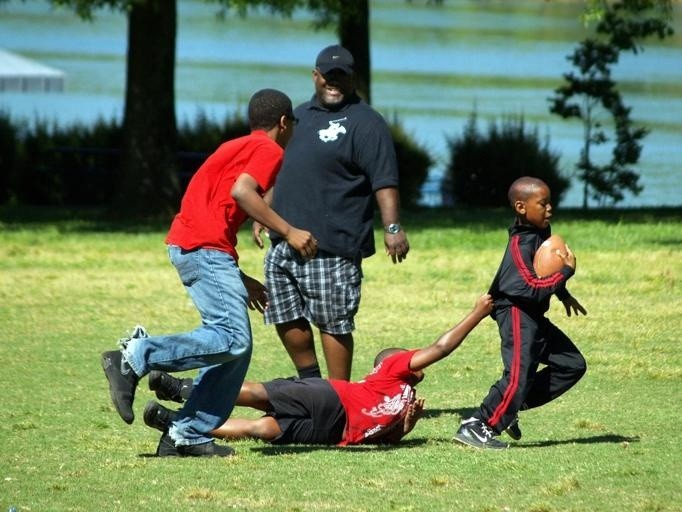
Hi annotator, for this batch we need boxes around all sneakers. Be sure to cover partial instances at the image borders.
[99,348,142,425]
[141,399,180,433]
[452,416,508,450]
[154,427,236,457]
[147,369,193,404]
[507,411,523,441]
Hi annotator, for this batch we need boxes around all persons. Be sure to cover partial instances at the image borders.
[453,177,587,450]
[101,90,320,456]
[145,291,495,444]
[250,45,411,381]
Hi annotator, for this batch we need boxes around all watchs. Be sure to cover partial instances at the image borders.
[382,223,402,234]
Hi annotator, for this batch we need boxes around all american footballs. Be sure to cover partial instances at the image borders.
[533,233,567,280]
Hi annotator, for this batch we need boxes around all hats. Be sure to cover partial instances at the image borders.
[314,43,356,75]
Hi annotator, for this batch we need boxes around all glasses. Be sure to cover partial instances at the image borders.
[288,117,298,125]
